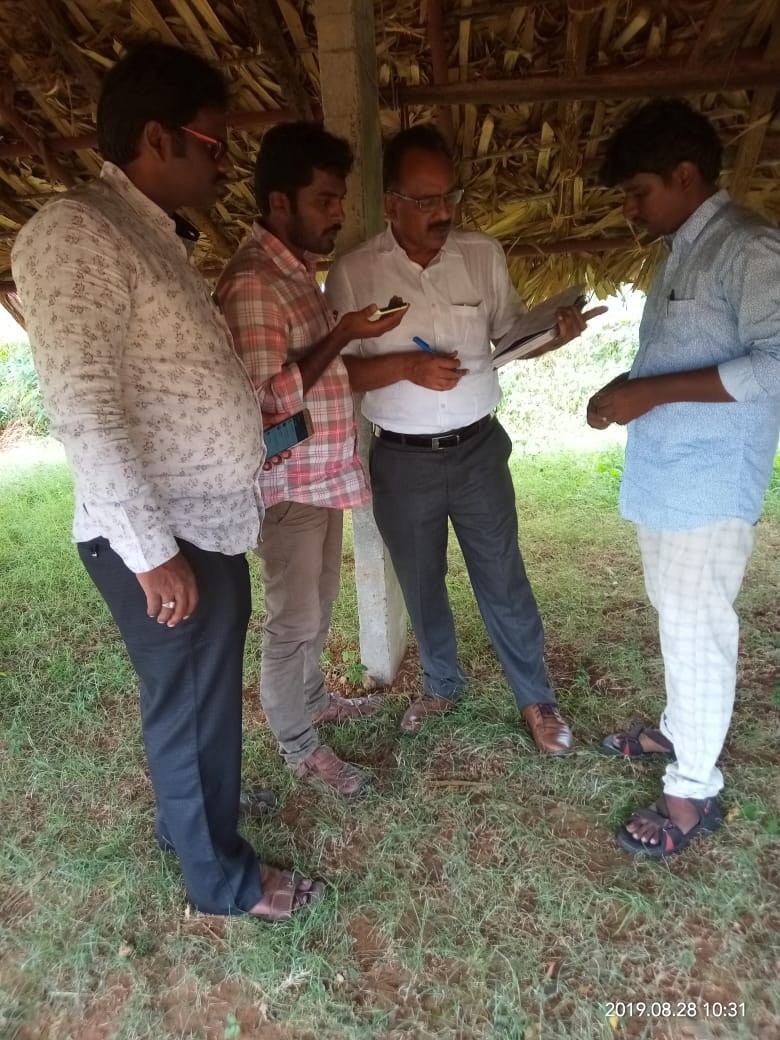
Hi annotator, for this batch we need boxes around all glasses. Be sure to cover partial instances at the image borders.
[181,126,228,161]
[388,189,465,213]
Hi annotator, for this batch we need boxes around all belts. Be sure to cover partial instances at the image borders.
[372,416,491,452]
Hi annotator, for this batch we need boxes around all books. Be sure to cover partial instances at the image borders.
[490,295,586,373]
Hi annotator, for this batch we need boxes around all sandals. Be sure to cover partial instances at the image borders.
[619,794,725,858]
[600,722,674,757]
[248,862,328,922]
[239,786,279,824]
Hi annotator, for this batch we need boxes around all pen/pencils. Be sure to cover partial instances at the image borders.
[412,336,460,374]
[669,289,675,300]
[333,310,338,322]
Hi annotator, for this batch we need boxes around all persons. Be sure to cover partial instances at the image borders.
[585,98,780,858]
[324,125,608,756]
[213,117,410,796]
[11,43,325,923]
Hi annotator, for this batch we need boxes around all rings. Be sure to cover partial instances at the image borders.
[162,601,175,609]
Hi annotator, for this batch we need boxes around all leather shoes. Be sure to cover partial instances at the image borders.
[523,702,572,755]
[314,694,383,725]
[294,744,369,797]
[400,691,463,733]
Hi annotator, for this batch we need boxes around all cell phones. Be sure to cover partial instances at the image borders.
[263,408,315,461]
[368,302,409,321]
